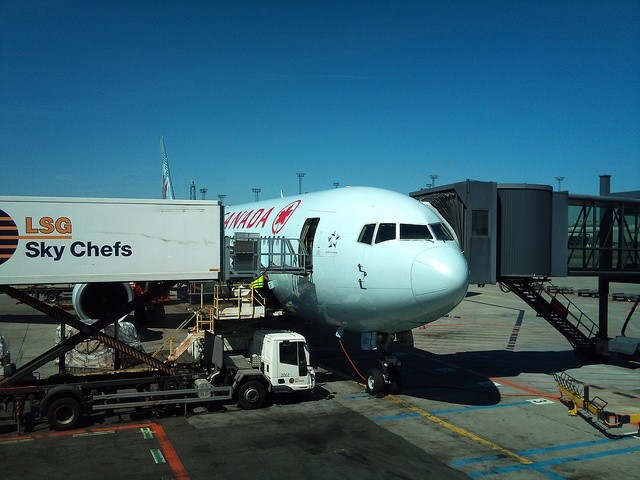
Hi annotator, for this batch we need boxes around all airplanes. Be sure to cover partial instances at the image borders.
[72,133,471,396]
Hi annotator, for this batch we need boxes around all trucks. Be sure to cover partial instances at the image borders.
[15,282,173,303]
[1,330,315,430]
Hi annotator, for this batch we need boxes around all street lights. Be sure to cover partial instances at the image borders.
[198,187,208,200]
[429,173,439,187]
[251,187,262,201]
[217,193,226,202]
[555,175,564,191]
[296,171,304,193]
